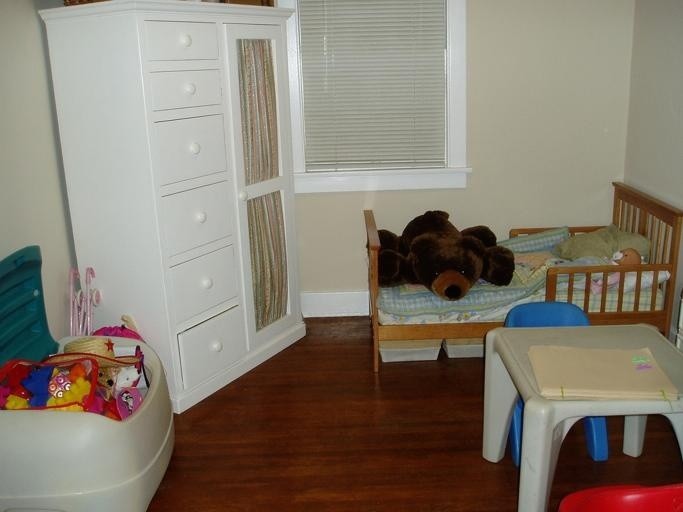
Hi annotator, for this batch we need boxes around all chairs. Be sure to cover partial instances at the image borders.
[504,302,609,467]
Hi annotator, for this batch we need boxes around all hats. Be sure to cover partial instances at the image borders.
[42,337,141,367]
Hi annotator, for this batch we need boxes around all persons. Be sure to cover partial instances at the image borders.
[555,247,641,295]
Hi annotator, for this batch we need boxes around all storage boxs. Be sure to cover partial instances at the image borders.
[0,246,175,511]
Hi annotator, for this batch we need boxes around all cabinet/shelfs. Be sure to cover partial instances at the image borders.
[38,4,307,415]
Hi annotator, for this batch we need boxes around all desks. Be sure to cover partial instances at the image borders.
[482,323,683,511]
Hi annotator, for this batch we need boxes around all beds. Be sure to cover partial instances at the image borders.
[363,181,683,373]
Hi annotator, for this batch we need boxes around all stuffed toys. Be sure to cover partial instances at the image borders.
[88,364,122,419]
[366,209,514,301]
